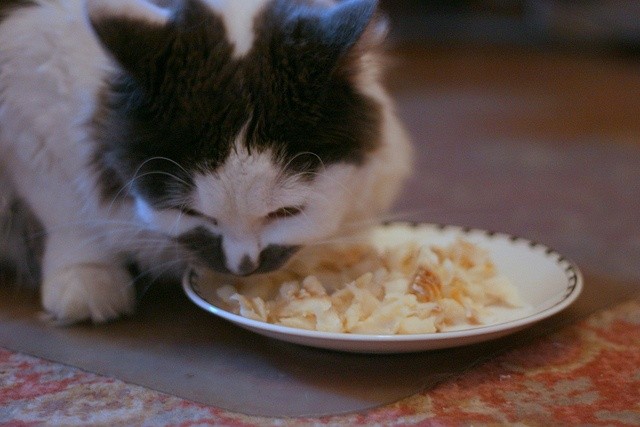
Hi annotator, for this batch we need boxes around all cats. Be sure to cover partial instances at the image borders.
[0,0,414,326]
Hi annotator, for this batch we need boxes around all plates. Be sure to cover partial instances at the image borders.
[182,221,584,354]
